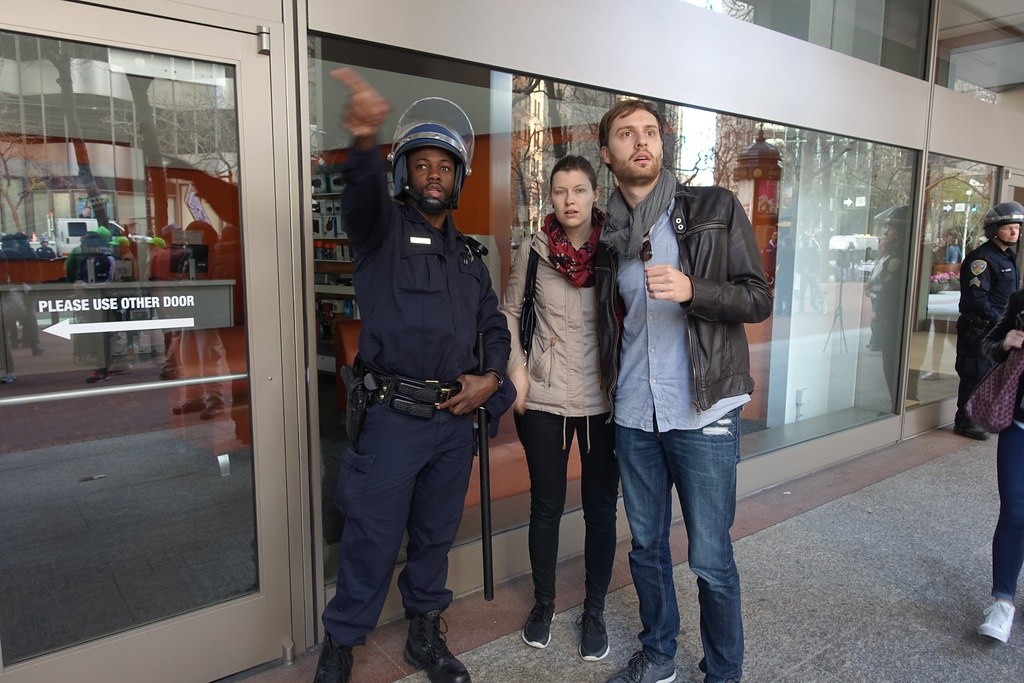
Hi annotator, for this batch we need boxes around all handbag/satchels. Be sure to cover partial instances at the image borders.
[520,234,537,364]
[964,345,1024,434]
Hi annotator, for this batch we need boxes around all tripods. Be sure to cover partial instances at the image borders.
[823,282,848,354]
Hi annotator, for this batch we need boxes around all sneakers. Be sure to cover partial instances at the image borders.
[521,599,555,648]
[606,651,677,683]
[978,601,1016,643]
[575,610,610,662]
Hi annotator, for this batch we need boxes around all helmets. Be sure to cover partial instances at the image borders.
[884,206,909,247]
[983,200,1024,240]
[392,123,467,210]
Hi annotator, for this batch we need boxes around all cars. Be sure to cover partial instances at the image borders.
[915,288,964,329]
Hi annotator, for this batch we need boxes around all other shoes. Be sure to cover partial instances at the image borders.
[32,345,45,354]
[159,371,183,381]
[953,425,991,440]
[87,366,132,384]
[173,401,225,421]
[922,370,941,380]
[2,373,17,383]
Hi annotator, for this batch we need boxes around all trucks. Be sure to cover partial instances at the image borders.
[27,212,133,259]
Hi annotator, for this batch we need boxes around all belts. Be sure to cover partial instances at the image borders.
[351,361,459,407]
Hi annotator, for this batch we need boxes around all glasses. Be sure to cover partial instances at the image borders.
[639,229,653,262]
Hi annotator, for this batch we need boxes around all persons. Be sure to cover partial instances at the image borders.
[595,100,774,683]
[502,155,618,660]
[761,230,815,314]
[978,289,1024,643]
[314,69,511,683]
[862,205,907,415]
[0,154,252,442]
[946,239,961,264]
[954,201,1024,439]
[80,207,91,217]
[920,320,957,379]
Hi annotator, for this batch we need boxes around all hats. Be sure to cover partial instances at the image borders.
[0,219,240,255]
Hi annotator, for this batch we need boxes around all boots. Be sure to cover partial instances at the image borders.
[313,625,354,683]
[404,608,472,683]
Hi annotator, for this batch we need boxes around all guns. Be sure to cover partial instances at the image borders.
[338,364,366,443]
[967,314,990,342]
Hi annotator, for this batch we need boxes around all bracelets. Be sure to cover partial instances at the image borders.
[492,372,503,385]
